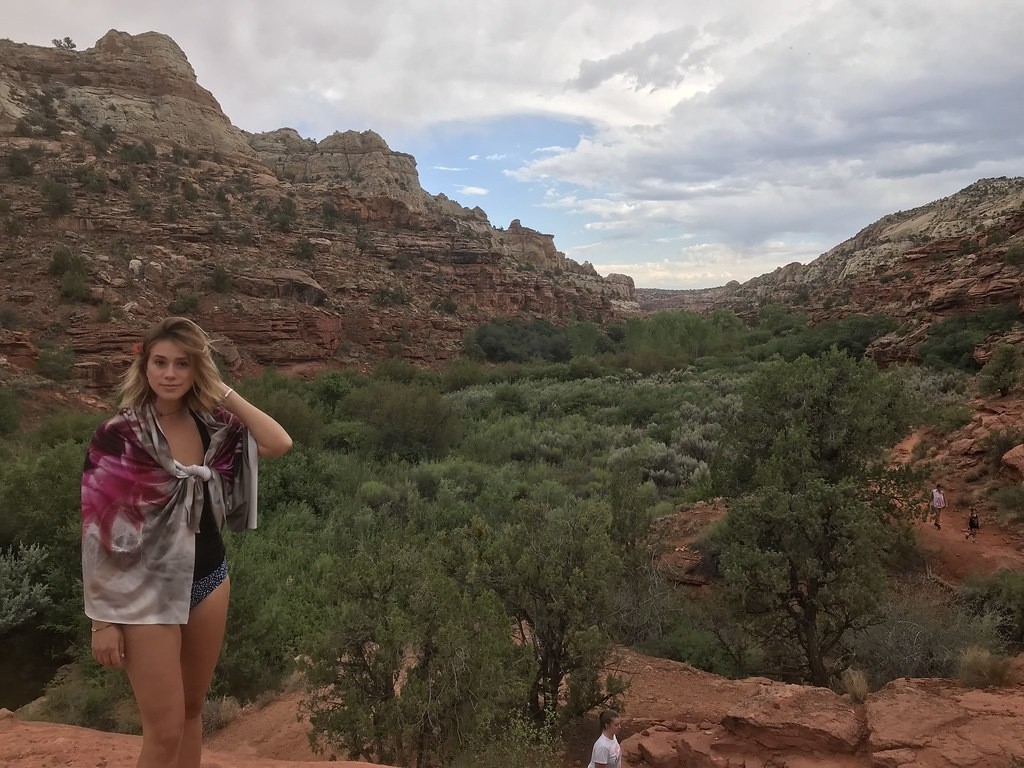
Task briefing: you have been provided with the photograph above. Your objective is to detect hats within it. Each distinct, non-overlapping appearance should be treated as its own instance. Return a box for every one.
[969,506,976,512]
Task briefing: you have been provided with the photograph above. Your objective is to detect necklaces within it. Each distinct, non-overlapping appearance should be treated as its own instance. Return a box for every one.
[155,407,184,416]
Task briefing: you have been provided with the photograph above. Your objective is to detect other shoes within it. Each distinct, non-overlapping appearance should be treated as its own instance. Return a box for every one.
[934,523,941,529]
[966,533,969,539]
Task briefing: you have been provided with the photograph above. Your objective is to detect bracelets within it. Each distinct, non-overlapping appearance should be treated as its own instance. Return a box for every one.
[91,624,112,632]
[225,388,233,397]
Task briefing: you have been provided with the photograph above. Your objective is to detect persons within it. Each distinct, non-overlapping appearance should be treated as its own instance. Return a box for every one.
[591,710,622,768]
[927,485,946,529]
[83,317,292,768]
[966,507,979,542]
